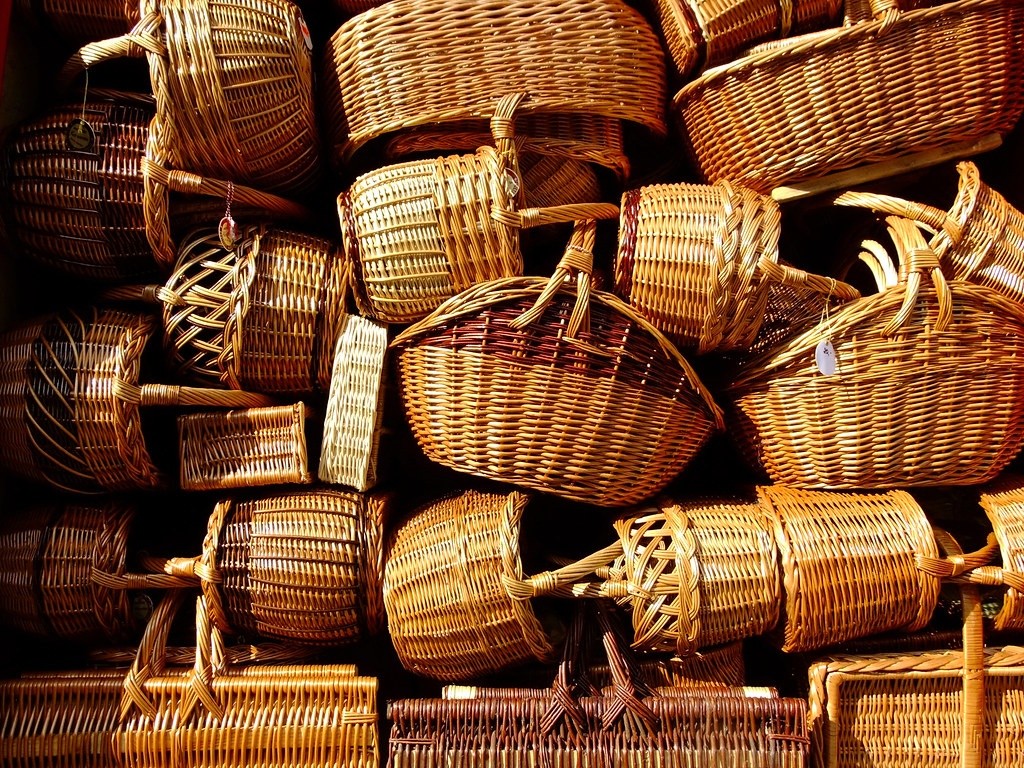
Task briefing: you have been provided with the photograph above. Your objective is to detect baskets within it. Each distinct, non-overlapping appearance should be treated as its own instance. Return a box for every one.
[0,1,1024,768]
